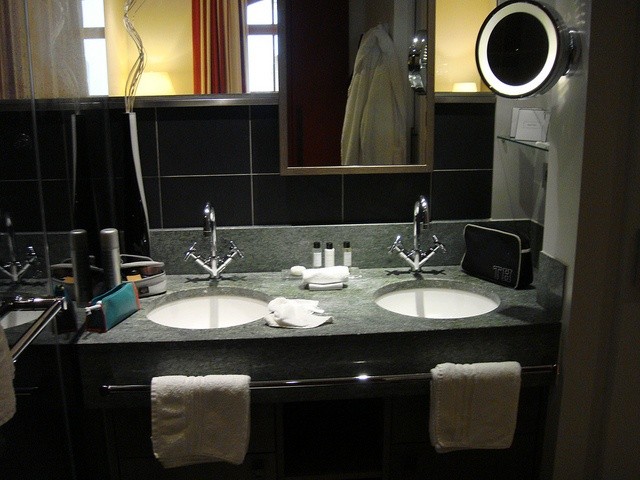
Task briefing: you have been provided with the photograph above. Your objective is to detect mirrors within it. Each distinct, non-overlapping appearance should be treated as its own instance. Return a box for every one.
[276,0,436,175]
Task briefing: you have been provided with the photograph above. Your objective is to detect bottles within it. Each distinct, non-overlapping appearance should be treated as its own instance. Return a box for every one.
[311,241,324,269]
[323,242,336,268]
[341,240,353,268]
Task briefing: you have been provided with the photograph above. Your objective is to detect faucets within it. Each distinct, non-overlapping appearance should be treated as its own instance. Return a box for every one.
[412,193,430,275]
[0,215,38,286]
[202,198,216,283]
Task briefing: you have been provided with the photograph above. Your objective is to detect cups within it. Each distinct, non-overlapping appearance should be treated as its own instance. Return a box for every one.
[98,228,122,292]
[69,229,94,308]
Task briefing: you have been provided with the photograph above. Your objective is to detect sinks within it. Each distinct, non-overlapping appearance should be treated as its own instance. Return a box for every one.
[370,279,501,320]
[145,286,272,328]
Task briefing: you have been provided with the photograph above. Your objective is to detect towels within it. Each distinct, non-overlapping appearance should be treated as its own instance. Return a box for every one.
[428,361,521,452]
[1,326,18,426]
[149,374,251,467]
[265,296,334,329]
[340,22,417,167]
